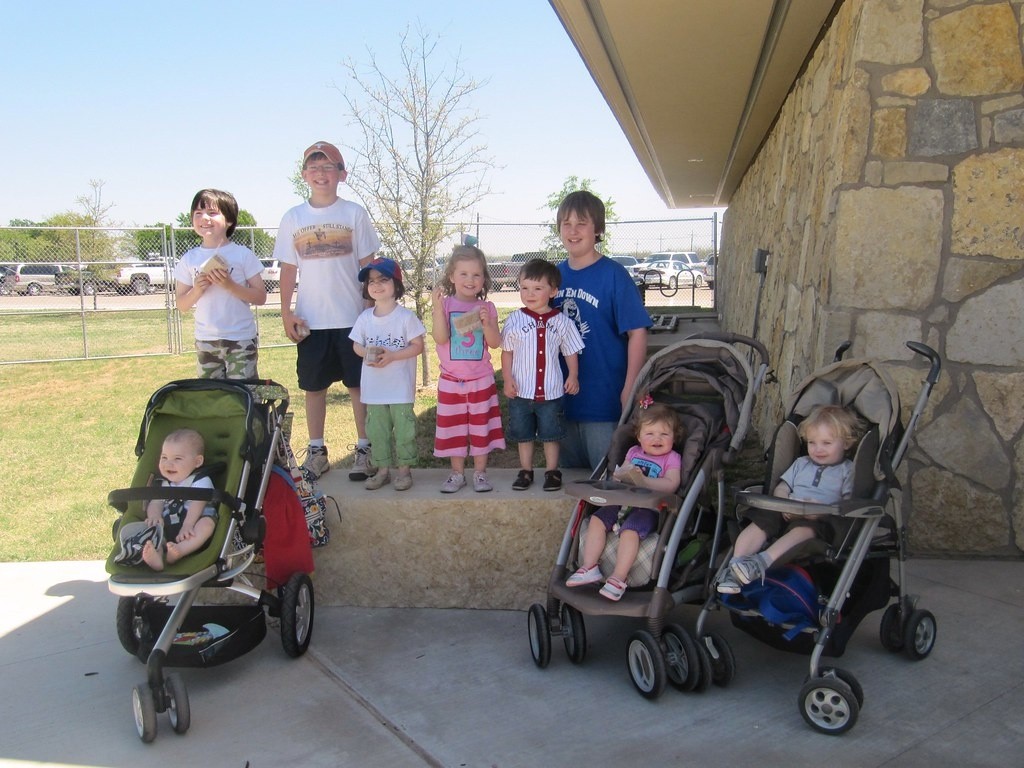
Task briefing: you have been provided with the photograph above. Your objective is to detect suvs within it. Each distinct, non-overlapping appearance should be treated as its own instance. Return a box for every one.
[632,251,703,276]
[258,257,299,293]
[704,253,719,290]
[610,255,640,279]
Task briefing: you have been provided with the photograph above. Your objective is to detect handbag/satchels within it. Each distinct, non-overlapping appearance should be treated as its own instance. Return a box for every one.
[274,429,333,546]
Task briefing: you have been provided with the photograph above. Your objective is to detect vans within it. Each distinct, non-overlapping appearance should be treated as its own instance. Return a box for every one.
[7,264,79,295]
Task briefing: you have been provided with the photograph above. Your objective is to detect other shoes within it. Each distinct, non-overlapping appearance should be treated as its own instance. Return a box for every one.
[599,575,626,602]
[565,566,603,587]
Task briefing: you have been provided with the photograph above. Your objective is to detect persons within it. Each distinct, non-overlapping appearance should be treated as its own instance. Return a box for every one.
[348,258,426,491]
[716,405,867,594]
[432,234,506,493]
[548,191,655,472]
[142,428,218,570]
[497,259,584,491]
[172,188,267,381]
[565,391,684,601]
[272,141,383,482]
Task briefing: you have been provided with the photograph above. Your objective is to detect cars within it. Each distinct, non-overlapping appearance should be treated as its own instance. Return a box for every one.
[0,266,17,295]
[399,258,443,291]
[638,261,704,290]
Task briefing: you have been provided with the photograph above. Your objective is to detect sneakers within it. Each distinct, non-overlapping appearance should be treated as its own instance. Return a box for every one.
[731,557,769,582]
[347,442,375,481]
[543,471,562,491]
[473,470,492,491]
[717,564,741,595]
[395,472,411,490]
[441,475,464,494]
[296,446,329,480]
[513,469,533,489]
[365,474,390,490]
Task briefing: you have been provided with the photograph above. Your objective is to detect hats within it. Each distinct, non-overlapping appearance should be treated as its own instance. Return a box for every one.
[358,256,402,282]
[302,141,345,170]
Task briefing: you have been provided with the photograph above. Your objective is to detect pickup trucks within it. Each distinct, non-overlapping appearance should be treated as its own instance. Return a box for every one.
[53,263,136,296]
[102,255,178,295]
[485,251,568,292]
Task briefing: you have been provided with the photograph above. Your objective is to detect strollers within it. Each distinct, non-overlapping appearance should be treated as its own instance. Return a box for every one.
[105,375,315,744]
[528,329,773,703]
[694,342,942,734]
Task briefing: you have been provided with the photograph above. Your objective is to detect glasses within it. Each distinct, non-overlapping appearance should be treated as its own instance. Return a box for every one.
[305,164,338,174]
